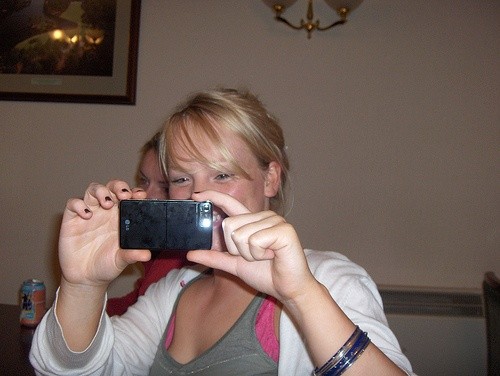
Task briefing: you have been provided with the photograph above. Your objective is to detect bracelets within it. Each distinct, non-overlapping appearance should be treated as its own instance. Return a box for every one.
[313,325,371,376]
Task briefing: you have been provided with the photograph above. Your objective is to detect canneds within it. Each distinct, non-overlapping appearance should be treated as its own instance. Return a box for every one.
[19,279,46,328]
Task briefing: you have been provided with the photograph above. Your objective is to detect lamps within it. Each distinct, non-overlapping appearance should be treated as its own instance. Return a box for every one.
[261,0,363,40]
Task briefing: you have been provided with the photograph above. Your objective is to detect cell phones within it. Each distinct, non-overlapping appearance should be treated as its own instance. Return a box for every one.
[118,199,213,250]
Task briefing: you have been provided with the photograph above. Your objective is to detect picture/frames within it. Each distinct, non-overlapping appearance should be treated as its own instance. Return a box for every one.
[0,0,142,105]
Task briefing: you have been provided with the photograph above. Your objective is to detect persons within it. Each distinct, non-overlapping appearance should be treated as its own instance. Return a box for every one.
[107,129,197,317]
[29,89,418,376]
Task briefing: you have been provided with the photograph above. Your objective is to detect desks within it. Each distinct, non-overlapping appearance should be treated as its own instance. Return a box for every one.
[0,304,36,376]
